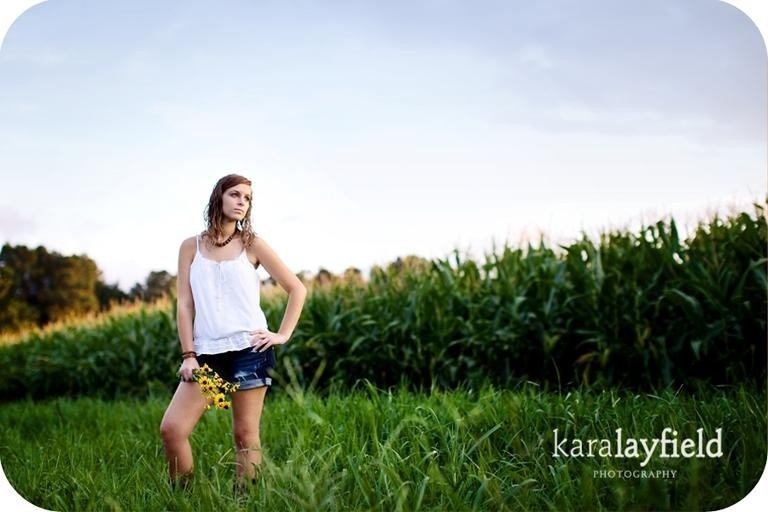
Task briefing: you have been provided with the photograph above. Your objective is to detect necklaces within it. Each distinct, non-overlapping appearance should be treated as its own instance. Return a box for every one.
[208,228,239,247]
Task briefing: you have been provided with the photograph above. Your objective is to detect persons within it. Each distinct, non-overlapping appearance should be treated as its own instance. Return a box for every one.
[160,175,307,495]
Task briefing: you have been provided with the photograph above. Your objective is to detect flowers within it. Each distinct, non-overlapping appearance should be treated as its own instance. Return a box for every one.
[177,359,241,412]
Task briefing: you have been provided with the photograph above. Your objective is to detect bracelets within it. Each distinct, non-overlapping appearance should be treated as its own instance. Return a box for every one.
[182,350,199,357]
